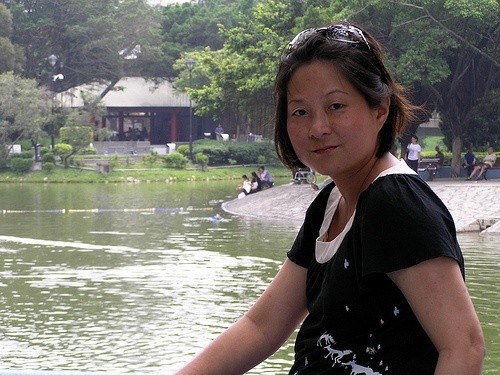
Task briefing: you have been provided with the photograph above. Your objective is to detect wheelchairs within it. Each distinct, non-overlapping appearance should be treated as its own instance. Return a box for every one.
[293,167,316,185]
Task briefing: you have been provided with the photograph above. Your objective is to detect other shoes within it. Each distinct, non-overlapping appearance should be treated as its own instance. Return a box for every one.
[465,177,473,181]
[474,177,482,181]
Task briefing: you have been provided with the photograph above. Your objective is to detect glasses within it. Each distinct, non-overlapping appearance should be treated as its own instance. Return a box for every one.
[282,24,374,60]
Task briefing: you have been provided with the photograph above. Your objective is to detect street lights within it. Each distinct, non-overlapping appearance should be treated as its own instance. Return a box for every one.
[47,52,59,151]
[184,59,196,160]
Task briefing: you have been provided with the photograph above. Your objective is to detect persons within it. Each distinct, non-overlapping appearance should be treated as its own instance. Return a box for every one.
[293,167,311,186]
[236,165,273,196]
[173,25,487,375]
[123,126,147,141]
[406,135,421,173]
[425,145,445,181]
[463,145,476,180]
[465,144,497,180]
[215,122,224,141]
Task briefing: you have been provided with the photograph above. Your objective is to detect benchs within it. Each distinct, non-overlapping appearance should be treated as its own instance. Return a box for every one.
[471,157,500,180]
[417,168,437,182]
